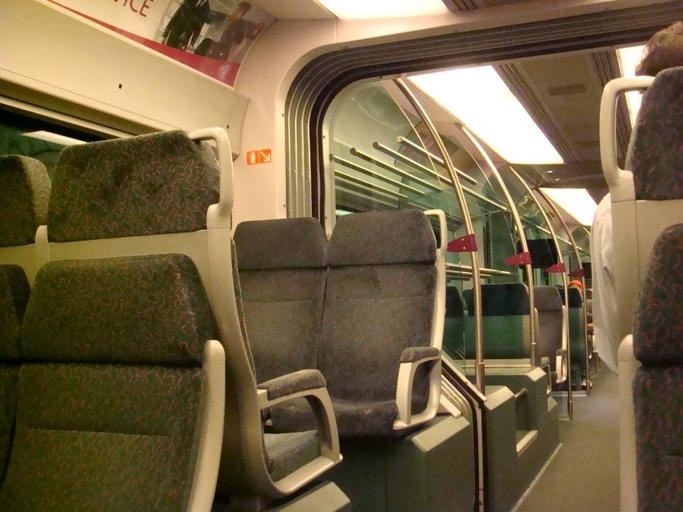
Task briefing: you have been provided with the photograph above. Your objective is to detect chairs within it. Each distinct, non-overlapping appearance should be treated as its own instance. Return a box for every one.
[2,126,448,512]
[448,283,599,512]
[600,74,683,510]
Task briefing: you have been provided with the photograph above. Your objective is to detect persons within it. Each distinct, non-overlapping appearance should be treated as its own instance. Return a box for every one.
[193,1,253,62]
[567,278,594,390]
[159,0,213,54]
[584,19,683,376]
